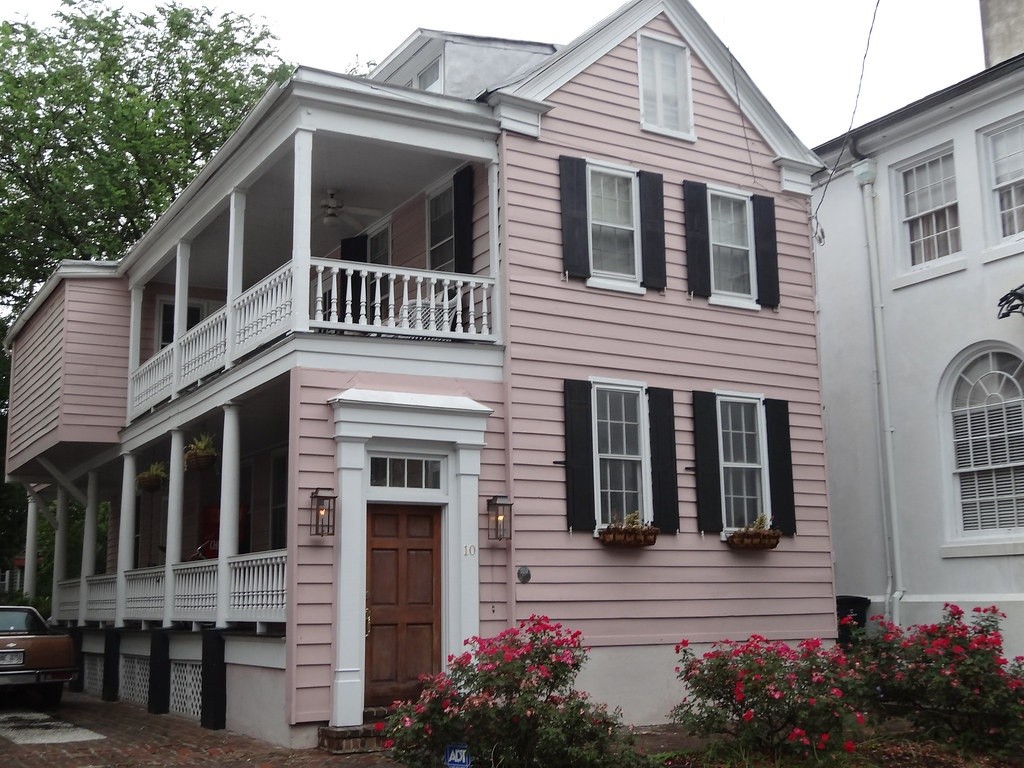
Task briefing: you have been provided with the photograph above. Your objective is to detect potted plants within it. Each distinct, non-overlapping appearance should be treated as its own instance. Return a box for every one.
[183,432,218,472]
[135,461,170,493]
[603,513,778,543]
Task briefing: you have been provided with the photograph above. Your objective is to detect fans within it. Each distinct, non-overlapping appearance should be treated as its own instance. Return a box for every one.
[283,189,383,232]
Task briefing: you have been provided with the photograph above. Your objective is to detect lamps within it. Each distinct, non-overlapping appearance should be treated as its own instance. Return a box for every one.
[487,496,514,540]
[309,488,338,537]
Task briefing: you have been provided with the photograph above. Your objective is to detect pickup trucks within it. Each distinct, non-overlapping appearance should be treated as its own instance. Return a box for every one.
[0,603,76,709]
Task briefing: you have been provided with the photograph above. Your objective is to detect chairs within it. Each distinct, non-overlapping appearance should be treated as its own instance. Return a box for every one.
[396,289,464,342]
